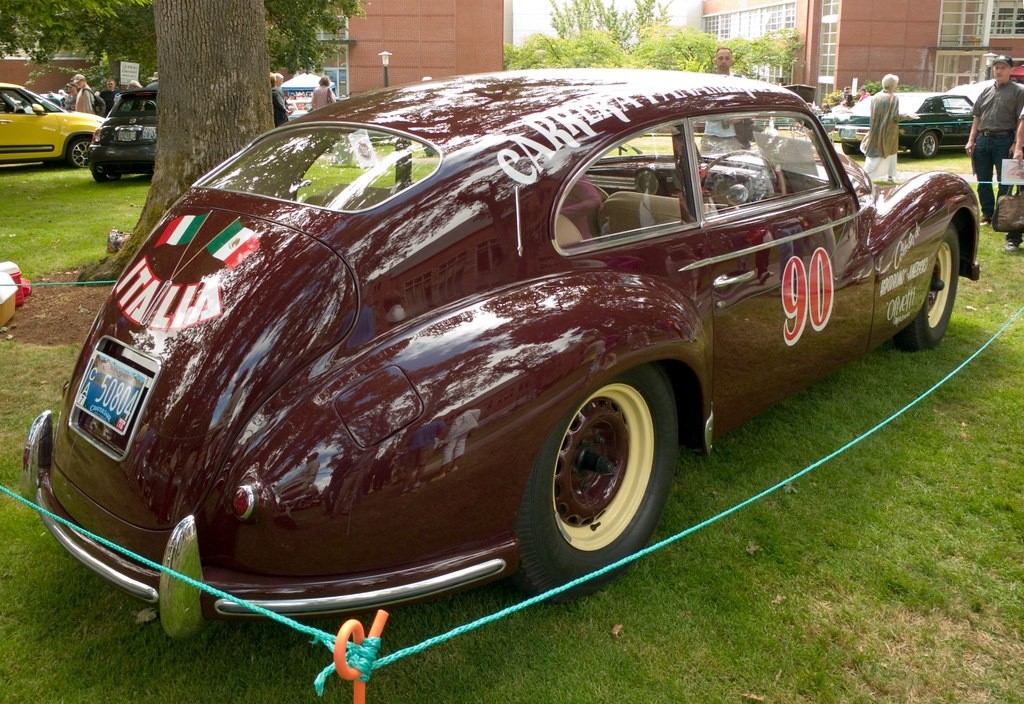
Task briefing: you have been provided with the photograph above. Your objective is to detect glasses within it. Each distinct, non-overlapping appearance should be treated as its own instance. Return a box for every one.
[66,86,71,88]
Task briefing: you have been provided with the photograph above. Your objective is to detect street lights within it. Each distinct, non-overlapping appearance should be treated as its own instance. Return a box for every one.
[378,51,393,88]
[983,51,998,80]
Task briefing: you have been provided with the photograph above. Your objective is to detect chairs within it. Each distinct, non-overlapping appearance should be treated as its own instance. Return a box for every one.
[317,181,389,212]
[554,215,583,248]
[598,190,714,230]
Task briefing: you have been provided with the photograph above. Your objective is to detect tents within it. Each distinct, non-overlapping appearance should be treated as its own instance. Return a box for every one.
[282,73,336,95]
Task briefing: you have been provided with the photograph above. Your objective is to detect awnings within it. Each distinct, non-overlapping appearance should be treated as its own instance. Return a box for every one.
[1009,67,1024,77]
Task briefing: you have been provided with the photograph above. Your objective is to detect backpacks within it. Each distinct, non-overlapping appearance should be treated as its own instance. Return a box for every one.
[82,88,106,116]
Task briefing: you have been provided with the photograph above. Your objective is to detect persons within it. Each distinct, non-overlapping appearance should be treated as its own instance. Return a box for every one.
[64,82,79,110]
[1002,108,1024,250]
[700,48,748,152]
[859,89,868,102]
[270,72,295,126]
[840,87,856,104]
[48,91,61,106]
[312,76,336,110]
[965,55,1024,226]
[58,89,67,106]
[100,80,120,116]
[73,74,95,114]
[864,74,921,180]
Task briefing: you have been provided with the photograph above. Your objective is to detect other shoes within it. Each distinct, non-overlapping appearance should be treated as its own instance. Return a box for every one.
[1004,236,1019,251]
[980,214,990,226]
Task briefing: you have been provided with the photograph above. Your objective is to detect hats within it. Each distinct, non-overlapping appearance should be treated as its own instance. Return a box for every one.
[152,72,158,80]
[992,54,1013,67]
[73,74,85,82]
[66,81,76,87]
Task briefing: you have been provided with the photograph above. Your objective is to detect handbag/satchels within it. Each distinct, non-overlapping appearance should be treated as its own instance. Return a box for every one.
[327,88,332,104]
[992,185,1024,233]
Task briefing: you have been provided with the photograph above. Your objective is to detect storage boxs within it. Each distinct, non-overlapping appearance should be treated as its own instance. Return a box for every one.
[0,272,19,328]
[0,261,25,306]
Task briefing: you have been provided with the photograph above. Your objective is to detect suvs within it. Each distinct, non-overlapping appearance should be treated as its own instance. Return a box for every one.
[88,87,158,183]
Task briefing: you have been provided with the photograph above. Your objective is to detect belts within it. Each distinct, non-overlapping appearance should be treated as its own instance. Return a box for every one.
[978,130,1011,137]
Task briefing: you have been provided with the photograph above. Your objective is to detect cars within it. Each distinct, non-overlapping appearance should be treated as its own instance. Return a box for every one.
[818,92,974,161]
[0,83,107,168]
[20,66,981,646]
